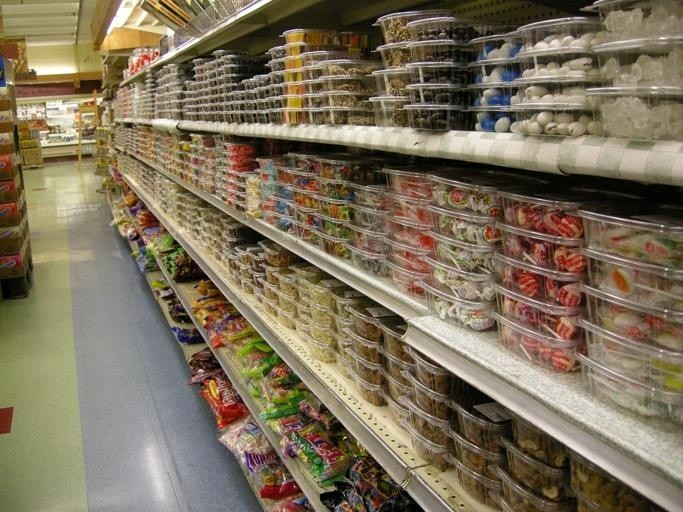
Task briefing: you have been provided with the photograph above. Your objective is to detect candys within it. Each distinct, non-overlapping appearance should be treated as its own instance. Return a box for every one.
[390,0,683,422]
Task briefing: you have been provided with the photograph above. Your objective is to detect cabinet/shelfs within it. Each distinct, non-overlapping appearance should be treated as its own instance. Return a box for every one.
[101,0,683,512]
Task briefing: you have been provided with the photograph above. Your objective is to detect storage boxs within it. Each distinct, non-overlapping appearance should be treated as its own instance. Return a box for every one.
[0,56,44,280]
